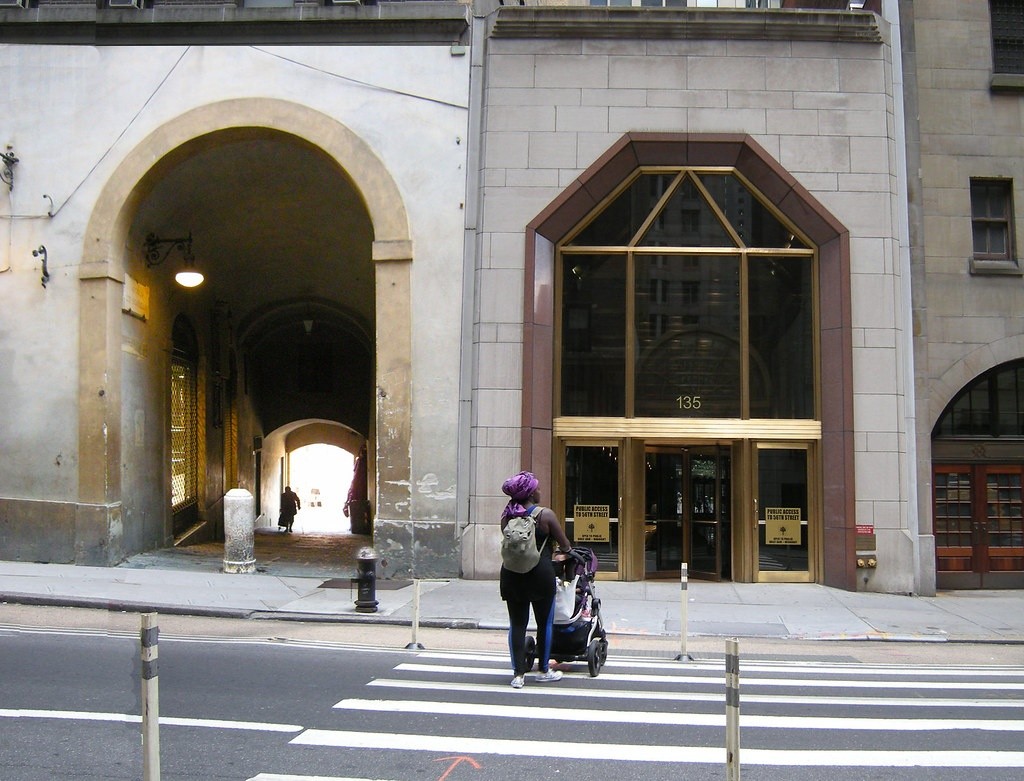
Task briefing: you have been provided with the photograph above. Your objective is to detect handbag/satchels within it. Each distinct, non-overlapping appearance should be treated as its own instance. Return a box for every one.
[554,574,579,622]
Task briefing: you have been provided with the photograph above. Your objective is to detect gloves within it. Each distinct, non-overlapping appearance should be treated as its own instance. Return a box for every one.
[564,547,586,567]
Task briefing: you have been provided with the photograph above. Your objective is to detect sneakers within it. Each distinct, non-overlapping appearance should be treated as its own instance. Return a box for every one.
[535,668,563,682]
[511,676,525,688]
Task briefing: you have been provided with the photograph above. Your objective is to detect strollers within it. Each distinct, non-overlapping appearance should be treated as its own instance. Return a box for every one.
[522,546,608,677]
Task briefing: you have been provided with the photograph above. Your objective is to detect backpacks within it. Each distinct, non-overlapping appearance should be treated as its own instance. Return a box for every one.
[501,507,549,573]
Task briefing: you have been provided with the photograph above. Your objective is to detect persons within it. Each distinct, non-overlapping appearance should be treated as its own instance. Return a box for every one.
[499,471,587,688]
[554,553,584,605]
[280,486,301,533]
